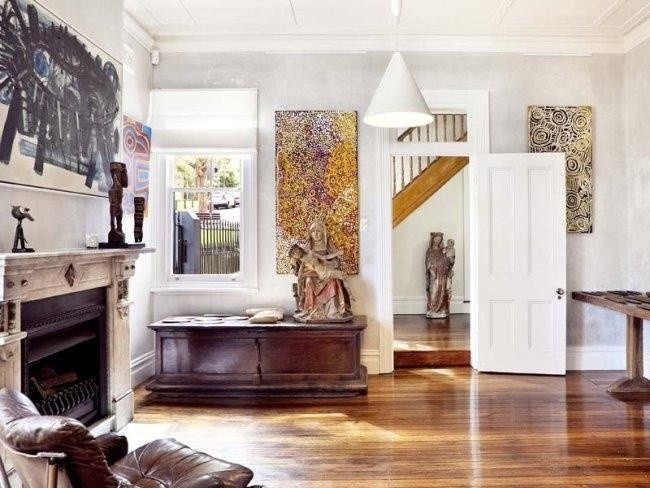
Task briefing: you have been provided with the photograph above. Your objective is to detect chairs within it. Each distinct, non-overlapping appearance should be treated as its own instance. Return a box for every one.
[0,387,262,488]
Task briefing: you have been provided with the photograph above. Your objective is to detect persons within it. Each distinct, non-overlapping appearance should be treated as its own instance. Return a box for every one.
[293,221,350,322]
[289,244,343,281]
[424,232,449,313]
[443,239,456,266]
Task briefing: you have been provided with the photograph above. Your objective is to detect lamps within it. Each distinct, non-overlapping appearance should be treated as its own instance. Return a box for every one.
[362,0,434,129]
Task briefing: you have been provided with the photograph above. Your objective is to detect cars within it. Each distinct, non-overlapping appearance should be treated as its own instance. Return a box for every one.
[208,186,241,208]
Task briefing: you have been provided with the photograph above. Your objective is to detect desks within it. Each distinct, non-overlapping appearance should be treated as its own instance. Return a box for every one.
[139,314,369,407]
[571,290,650,402]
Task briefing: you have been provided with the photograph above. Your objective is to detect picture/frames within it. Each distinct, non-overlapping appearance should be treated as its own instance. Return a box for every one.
[0,0,123,199]
